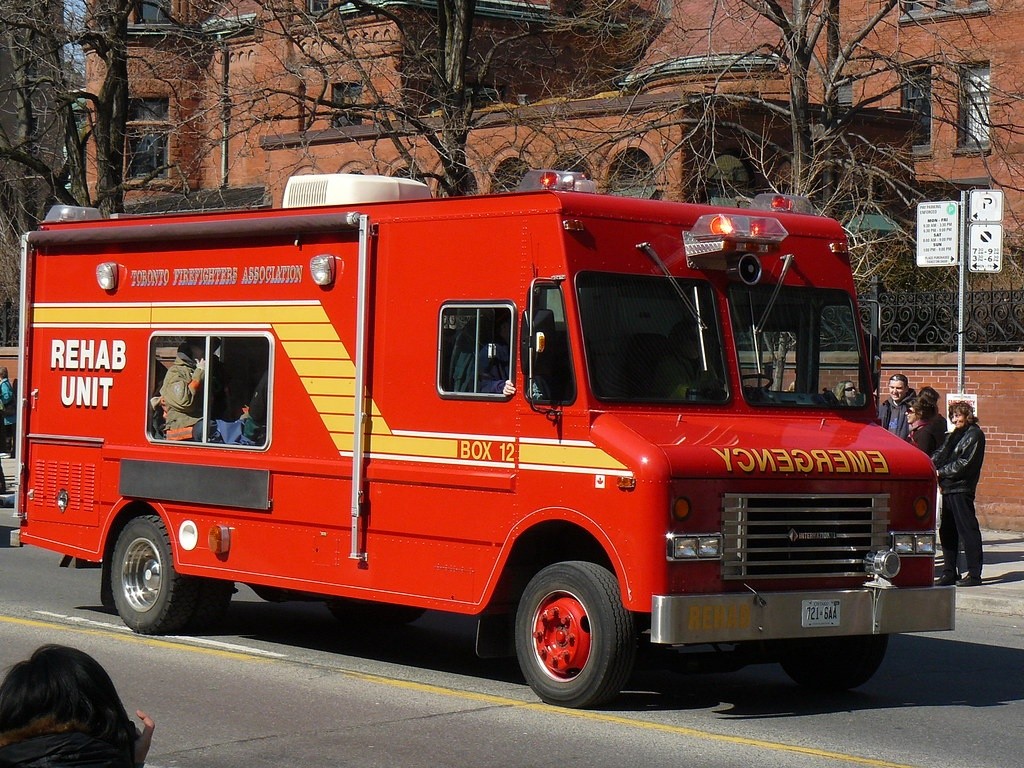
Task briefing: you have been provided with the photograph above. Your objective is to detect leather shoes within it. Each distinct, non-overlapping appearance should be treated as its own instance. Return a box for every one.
[934,575,961,586]
[956,575,982,587]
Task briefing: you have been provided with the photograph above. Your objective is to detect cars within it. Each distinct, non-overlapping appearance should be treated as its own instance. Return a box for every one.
[0,490,15,507]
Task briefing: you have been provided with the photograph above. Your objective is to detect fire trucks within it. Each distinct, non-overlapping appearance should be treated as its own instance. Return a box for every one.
[10,167,953,707]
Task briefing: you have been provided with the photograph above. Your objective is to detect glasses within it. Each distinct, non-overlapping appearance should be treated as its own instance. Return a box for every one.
[907,409,915,413]
[845,386,855,392]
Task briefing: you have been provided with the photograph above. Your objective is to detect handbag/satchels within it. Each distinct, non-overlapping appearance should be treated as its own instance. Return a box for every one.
[0,383,16,417]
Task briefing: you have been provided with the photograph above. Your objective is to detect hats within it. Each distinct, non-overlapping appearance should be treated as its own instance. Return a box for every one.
[177,336,221,353]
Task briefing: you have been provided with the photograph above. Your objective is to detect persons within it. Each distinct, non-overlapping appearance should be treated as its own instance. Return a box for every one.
[932,401,986,587]
[159,338,205,442]
[634,312,727,402]
[0,367,17,459]
[472,317,516,396]
[784,379,796,393]
[0,643,155,768]
[878,374,916,444]
[832,380,866,407]
[905,386,948,460]
[210,348,268,445]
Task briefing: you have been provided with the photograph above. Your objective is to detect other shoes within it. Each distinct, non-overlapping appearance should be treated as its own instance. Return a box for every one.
[0,452,11,459]
